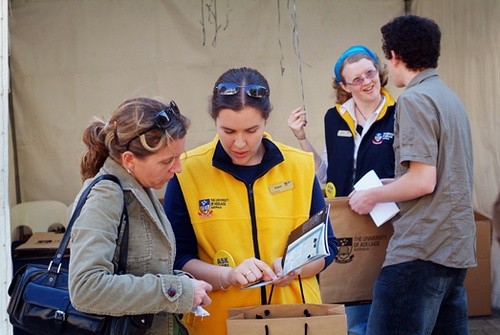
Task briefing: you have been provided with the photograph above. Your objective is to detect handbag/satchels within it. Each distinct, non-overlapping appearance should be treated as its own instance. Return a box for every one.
[7,263,153,335]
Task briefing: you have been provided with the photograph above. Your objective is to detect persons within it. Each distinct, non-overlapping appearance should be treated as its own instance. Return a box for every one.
[163,69,338,335]
[69,98,212,335]
[285,44,397,198]
[348,14,478,335]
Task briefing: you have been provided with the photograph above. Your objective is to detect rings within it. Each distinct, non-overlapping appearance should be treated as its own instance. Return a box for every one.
[244,271,252,277]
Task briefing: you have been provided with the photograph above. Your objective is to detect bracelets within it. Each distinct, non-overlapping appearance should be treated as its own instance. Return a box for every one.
[296,136,306,140]
[217,267,232,291]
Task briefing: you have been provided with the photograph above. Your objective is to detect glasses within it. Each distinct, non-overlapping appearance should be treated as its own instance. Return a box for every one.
[214,82,268,99]
[344,64,378,87]
[126,100,180,152]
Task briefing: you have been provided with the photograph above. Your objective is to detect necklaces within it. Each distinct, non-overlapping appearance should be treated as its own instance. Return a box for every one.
[354,97,383,121]
